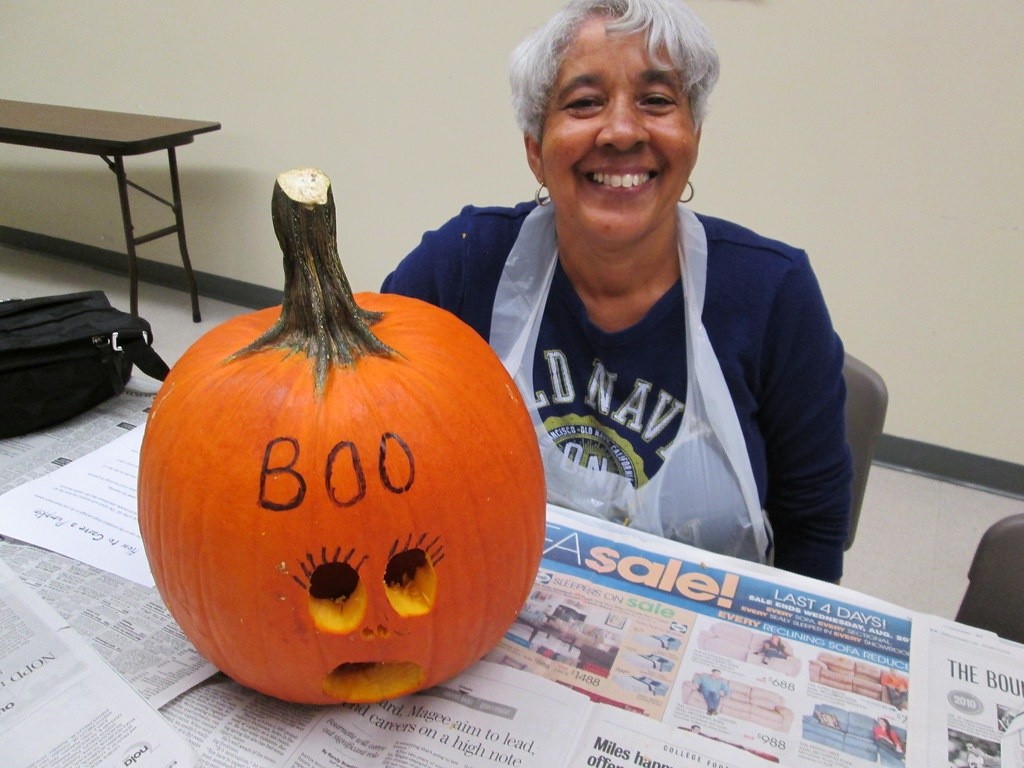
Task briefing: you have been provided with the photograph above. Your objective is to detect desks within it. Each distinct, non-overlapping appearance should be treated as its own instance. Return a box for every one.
[0,96,224,328]
[2,353,1024,767]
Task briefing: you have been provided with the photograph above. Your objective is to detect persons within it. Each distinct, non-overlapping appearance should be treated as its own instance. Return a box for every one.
[881,666,910,709]
[695,666,730,716]
[959,742,994,768]
[874,718,905,767]
[754,634,791,667]
[691,725,701,734]
[375,0,856,589]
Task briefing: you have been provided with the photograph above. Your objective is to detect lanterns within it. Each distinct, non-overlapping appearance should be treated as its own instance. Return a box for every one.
[136,166,550,708]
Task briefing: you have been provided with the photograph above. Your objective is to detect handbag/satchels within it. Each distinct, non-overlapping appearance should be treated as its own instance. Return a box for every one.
[0,289,173,439]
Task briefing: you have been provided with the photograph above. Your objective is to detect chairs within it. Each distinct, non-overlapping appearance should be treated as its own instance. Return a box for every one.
[843,354,888,603]
[979,615,985,618]
[953,512,1024,647]
